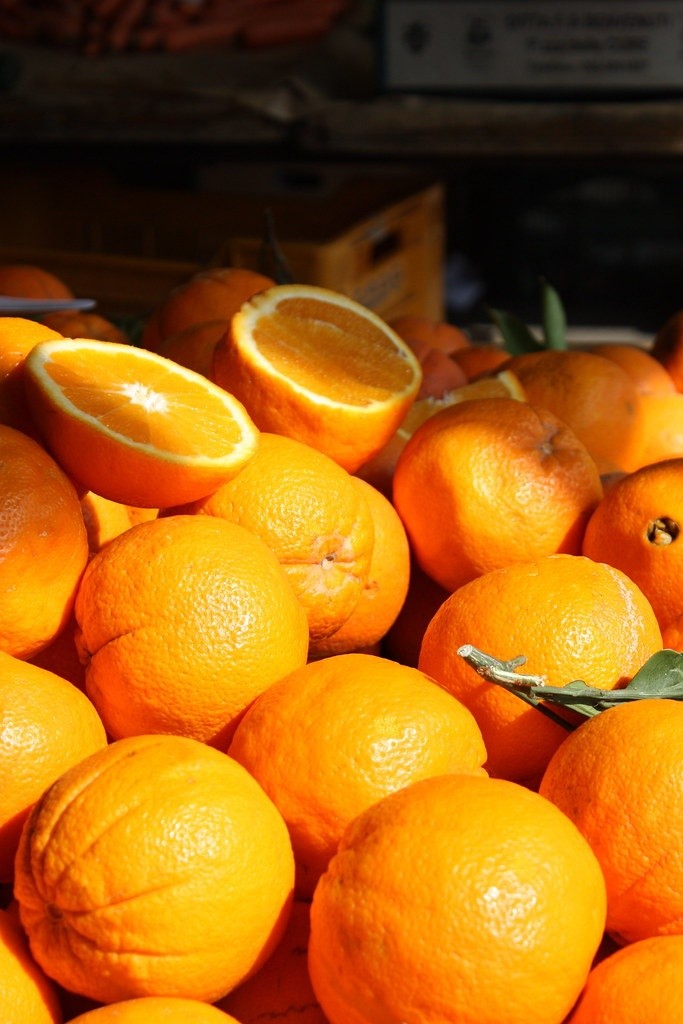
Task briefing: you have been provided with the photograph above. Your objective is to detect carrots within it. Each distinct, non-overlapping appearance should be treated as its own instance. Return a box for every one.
[0,0,351,51]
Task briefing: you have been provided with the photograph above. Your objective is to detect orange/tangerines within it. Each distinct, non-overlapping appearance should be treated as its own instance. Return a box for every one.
[0,267,683,1024]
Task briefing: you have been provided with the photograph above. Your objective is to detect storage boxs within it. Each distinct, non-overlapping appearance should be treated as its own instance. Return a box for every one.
[0,161,444,328]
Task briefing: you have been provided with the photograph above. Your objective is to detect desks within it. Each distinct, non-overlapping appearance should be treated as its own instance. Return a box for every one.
[0,91,683,329]
